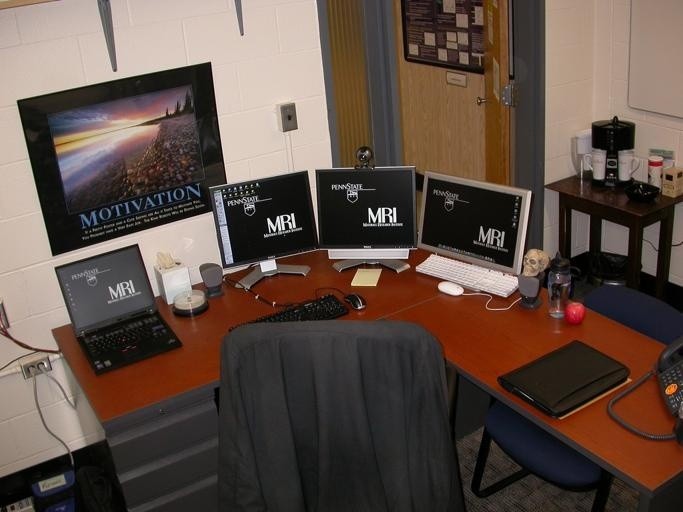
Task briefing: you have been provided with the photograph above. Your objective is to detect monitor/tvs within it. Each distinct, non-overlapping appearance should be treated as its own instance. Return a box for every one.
[315,166,417,274]
[417,171,533,275]
[209,170,319,289]
[17,62,227,257]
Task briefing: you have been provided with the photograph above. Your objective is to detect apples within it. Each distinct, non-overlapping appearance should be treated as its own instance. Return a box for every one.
[565,302,586,325]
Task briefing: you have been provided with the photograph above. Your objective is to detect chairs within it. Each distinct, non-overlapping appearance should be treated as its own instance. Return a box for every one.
[468,281,683,510]
[216,316,454,512]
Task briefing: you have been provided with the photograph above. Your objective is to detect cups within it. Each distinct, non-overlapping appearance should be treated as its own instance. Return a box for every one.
[619,150,640,182]
[584,150,606,180]
[647,156,663,187]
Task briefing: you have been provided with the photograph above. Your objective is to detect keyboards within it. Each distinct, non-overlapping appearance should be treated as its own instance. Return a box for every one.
[415,254,519,298]
[229,293,349,333]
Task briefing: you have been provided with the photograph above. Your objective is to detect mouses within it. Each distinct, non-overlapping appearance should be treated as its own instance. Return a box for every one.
[345,295,366,310]
[438,281,464,296]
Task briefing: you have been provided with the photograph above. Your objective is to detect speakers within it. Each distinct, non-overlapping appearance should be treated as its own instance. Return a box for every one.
[199,263,223,297]
[519,276,543,308]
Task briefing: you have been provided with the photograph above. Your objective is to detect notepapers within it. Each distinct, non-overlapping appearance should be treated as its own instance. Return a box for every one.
[350,268,384,287]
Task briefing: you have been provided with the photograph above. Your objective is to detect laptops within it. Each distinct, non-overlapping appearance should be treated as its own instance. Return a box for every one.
[54,244,182,375]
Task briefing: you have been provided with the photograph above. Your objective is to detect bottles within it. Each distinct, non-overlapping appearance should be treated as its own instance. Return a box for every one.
[547,252,582,318]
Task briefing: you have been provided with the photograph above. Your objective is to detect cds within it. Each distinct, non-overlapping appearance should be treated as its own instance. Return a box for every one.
[172,290,208,317]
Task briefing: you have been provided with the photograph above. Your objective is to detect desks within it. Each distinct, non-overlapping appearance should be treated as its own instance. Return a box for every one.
[48,246,683,511]
[544,170,682,301]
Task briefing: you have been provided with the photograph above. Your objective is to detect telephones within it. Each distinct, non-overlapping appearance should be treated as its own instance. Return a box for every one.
[656,336,683,416]
